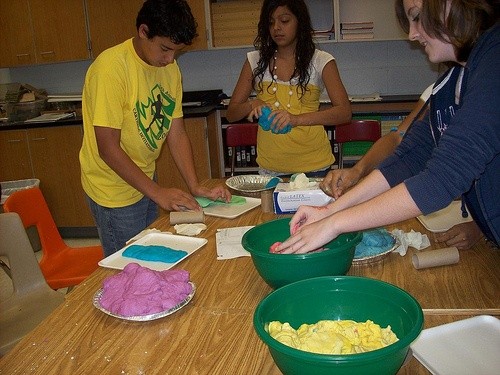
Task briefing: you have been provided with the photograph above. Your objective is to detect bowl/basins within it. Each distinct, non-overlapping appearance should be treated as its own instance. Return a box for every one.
[241,217,363,289]
[252,276,425,375]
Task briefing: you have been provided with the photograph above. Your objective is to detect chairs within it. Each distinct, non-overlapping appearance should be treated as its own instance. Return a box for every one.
[3,187,104,290]
[226,123,258,177]
[335,120,381,169]
[0,212,64,358]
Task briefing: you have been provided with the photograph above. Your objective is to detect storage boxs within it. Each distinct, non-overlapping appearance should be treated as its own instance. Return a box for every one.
[0,97,49,122]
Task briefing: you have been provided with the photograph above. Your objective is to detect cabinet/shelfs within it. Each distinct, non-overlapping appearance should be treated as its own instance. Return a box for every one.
[0,0,90,68]
[0,107,99,239]
[156,105,220,217]
[206,0,409,50]
[84,0,208,59]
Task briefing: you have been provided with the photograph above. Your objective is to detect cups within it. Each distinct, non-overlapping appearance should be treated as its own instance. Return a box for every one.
[261,190,273,213]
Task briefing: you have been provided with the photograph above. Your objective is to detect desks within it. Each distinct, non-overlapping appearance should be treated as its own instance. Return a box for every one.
[64,178,500,315]
[0,301,500,375]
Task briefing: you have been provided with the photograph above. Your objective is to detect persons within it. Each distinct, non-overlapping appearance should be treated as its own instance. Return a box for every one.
[318,82,483,250]
[275,0,500,254]
[79,0,233,258]
[227,0,351,178]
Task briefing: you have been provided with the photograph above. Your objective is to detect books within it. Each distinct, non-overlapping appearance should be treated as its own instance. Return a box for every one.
[340,21,374,39]
[311,30,331,40]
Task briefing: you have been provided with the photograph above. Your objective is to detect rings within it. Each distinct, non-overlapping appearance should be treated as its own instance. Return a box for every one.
[325,187,329,191]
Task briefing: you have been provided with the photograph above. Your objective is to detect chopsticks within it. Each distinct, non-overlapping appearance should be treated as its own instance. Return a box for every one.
[421,308,500,315]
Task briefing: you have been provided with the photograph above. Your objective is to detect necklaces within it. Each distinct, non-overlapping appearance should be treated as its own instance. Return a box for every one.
[273,49,293,110]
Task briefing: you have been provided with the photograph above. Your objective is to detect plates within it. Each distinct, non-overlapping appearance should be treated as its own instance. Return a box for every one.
[97,233,208,272]
[187,197,261,218]
[352,231,402,264]
[409,315,500,375]
[93,281,197,321]
[416,199,474,232]
[226,175,284,196]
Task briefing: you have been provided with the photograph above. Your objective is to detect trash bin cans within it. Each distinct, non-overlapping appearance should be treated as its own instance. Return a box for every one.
[0,179,42,252]
